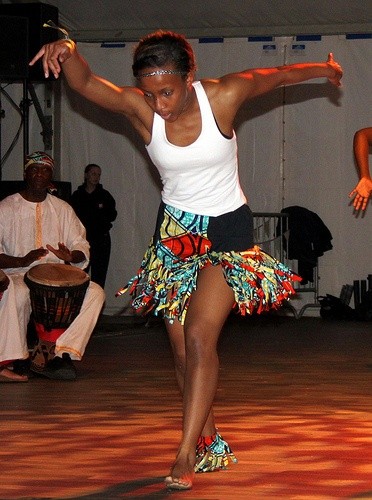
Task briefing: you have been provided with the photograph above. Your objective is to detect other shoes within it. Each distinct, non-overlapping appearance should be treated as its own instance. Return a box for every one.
[0,369,28,382]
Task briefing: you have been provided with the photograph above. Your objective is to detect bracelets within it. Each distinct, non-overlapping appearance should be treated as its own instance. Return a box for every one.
[43,20,75,52]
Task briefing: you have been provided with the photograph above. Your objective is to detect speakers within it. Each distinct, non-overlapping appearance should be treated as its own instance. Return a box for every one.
[0,1,59,84]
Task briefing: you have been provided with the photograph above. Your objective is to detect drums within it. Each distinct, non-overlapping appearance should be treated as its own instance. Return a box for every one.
[22,261,90,377]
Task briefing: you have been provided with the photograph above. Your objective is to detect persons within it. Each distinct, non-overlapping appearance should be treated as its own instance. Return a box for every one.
[348,127,372,211]
[0,150,108,382]
[29,31,346,491]
[68,163,118,337]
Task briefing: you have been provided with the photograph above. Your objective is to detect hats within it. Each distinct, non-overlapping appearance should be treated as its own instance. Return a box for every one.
[24,151,56,173]
